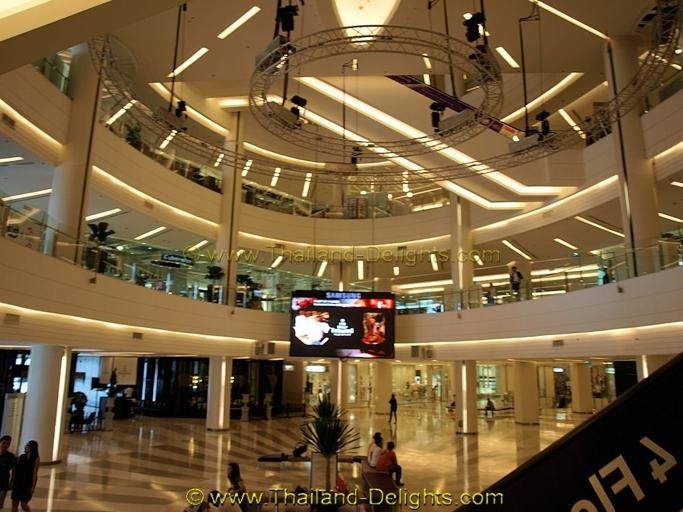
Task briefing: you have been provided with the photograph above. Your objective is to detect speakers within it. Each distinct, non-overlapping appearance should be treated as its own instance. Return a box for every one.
[409,346,423,359]
[265,343,275,354]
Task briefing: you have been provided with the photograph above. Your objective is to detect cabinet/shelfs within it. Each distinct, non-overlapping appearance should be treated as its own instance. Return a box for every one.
[98,397,136,420]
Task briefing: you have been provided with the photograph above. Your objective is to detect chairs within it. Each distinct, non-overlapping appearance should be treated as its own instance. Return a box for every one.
[84,411,96,430]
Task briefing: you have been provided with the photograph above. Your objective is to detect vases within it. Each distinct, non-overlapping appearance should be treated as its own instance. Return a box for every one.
[64,398,74,434]
[242,394,250,421]
[264,393,273,420]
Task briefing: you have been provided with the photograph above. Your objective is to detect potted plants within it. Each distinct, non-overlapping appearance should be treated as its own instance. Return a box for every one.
[87,221,116,274]
[306,388,361,508]
[204,265,253,308]
[104,368,116,431]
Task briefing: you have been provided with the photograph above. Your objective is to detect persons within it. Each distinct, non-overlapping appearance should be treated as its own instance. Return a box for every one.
[108,367,118,387]
[0,435,16,510]
[601,267,610,284]
[366,432,382,461]
[205,490,242,511]
[485,283,496,304]
[13,438,40,511]
[388,424,397,449]
[368,436,383,468]
[374,440,404,486]
[509,264,523,300]
[482,397,495,415]
[225,461,247,509]
[386,393,397,423]
[447,394,456,412]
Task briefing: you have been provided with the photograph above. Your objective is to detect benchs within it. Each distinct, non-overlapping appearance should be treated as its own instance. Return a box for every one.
[441,405,516,419]
[258,451,402,509]
